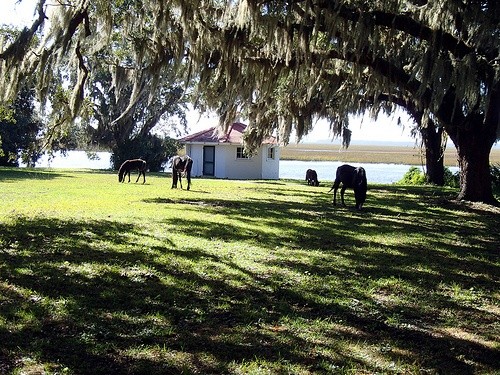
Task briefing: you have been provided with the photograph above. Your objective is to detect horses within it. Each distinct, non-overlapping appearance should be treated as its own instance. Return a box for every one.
[117,158,147,185]
[170,155,193,191]
[305,169,318,187]
[328,164,368,211]
